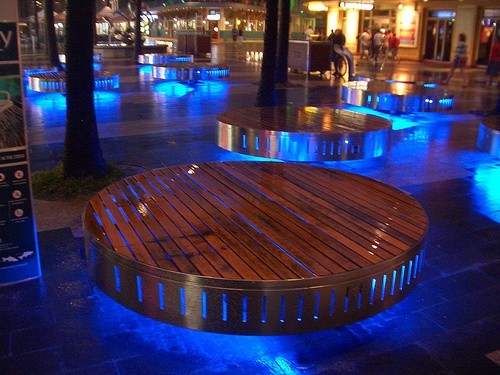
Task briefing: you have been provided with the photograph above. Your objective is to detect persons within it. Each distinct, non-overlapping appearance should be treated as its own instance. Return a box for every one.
[359,27,401,62]
[328,29,345,77]
[232,27,237,48]
[315,29,323,41]
[486,37,500,86]
[239,28,243,49]
[443,33,469,87]
[305,26,314,41]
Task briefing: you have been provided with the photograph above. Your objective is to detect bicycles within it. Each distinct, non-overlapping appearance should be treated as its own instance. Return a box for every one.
[331,49,347,76]
